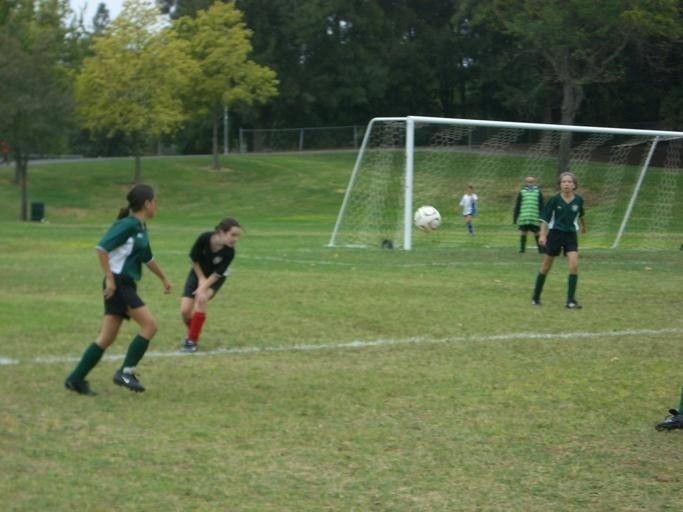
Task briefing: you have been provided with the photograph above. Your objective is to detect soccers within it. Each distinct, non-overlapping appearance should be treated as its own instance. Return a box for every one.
[413,206,442,232]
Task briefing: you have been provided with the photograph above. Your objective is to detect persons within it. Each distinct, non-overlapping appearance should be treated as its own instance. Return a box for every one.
[653,378,683,433]
[511,177,547,257]
[176,217,245,352]
[529,172,586,310]
[453,185,477,238]
[61,184,176,398]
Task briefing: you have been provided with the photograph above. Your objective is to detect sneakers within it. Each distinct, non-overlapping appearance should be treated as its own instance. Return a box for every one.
[565,300,583,309]
[654,408,683,432]
[181,338,199,352]
[113,368,148,394]
[530,298,541,305]
[64,376,99,397]
